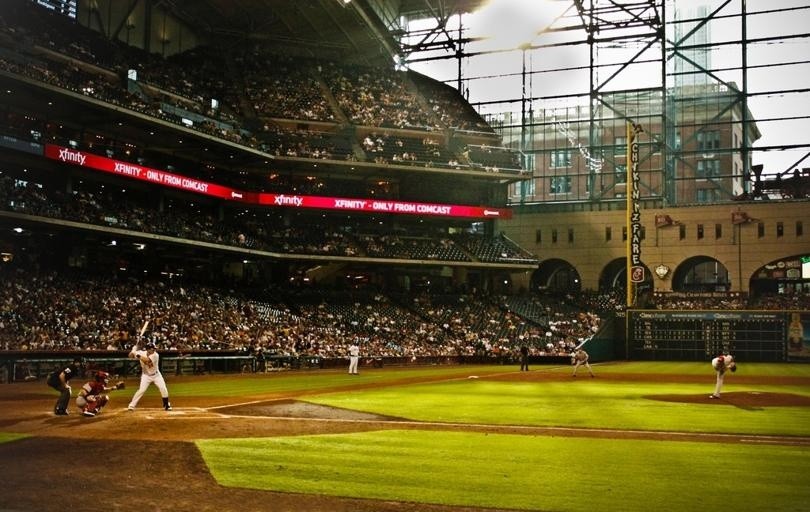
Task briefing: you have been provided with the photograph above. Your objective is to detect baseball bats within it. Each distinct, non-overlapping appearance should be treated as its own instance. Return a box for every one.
[134,321,150,345]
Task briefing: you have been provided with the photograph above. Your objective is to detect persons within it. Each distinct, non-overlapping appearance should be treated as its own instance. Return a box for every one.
[707,353,736,399]
[122,341,172,410]
[45,356,90,416]
[75,370,125,417]
[1,1,608,384]
[609,281,810,318]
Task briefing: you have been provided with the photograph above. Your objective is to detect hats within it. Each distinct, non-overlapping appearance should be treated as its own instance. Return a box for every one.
[144,341,159,350]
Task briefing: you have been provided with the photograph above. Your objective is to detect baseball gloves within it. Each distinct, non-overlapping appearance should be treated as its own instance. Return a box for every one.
[117,382,125,389]
[730,363,736,372]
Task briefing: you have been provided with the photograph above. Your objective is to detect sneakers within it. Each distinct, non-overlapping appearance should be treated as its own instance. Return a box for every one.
[53,406,100,417]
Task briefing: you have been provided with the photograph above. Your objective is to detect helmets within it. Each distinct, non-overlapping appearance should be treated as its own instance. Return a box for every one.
[94,371,110,383]
